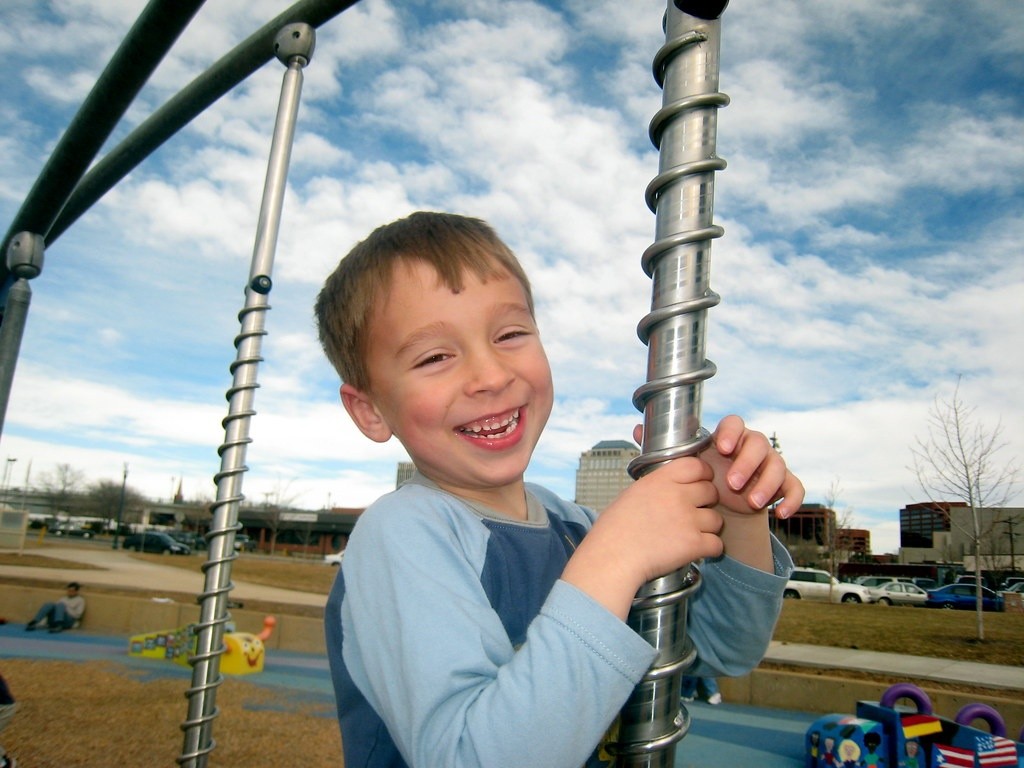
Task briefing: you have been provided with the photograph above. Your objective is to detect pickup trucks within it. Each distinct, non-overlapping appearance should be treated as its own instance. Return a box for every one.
[853,574,935,589]
[233,535,258,553]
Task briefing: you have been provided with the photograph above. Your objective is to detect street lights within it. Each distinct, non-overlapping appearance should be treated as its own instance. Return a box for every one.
[1,458,17,481]
[113,459,131,551]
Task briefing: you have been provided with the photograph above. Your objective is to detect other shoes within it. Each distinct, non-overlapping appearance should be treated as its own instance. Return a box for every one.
[26,621,36,630]
[50,624,63,632]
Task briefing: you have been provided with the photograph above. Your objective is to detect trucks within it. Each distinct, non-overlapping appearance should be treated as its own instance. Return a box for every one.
[28,512,134,536]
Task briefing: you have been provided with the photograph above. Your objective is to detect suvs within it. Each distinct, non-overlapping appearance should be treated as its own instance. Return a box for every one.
[1002,577,1024,588]
[952,573,988,594]
[784,567,872,606]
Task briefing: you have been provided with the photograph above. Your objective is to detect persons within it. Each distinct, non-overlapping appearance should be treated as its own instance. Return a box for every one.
[944,567,956,584]
[0,674,18,768]
[25,582,86,632]
[314,212,804,768]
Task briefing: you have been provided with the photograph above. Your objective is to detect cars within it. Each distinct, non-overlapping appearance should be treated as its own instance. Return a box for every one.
[926,582,1005,612]
[324,550,346,567]
[123,532,191,556]
[867,580,927,607]
[168,530,207,552]
[49,521,95,540]
[1007,582,1024,592]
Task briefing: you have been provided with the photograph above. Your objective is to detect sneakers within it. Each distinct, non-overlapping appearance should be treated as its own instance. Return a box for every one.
[681,697,694,703]
[708,693,722,704]
